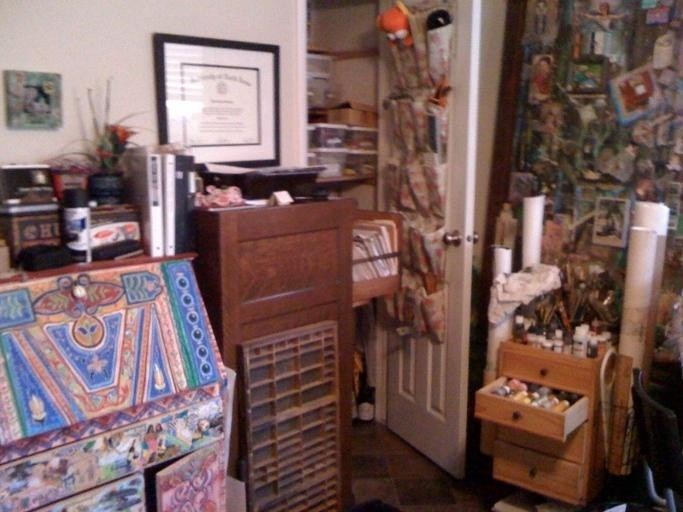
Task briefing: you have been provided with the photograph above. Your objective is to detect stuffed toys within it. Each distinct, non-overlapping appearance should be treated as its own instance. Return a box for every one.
[374,4,414,48]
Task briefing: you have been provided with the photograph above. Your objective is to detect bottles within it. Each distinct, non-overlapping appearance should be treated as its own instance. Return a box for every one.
[61,187,91,265]
[513,312,612,362]
[492,377,578,413]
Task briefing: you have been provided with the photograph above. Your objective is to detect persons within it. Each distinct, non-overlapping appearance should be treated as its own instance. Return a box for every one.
[580,3,628,30]
[23,81,52,117]
[531,55,550,96]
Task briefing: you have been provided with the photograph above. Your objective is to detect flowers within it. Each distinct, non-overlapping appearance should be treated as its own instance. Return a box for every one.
[46,76,146,173]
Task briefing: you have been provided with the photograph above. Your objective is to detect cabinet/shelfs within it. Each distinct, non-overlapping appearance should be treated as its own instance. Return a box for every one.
[186,189,357,512]
[475,333,620,512]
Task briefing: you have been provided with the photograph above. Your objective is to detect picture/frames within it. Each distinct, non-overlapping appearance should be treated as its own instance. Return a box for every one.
[4,71,60,128]
[153,32,281,168]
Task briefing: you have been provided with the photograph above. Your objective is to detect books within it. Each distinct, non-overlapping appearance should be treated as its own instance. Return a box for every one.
[353,215,401,283]
[129,152,164,263]
[173,153,189,255]
[144,152,180,258]
[188,155,196,253]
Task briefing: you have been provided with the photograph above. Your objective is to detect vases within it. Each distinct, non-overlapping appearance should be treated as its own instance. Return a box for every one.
[88,171,125,206]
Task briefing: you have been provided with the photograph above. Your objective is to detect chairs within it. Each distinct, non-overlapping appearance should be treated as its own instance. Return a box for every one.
[631,367,683,512]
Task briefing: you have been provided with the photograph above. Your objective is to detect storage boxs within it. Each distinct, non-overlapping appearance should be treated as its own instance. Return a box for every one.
[306,99,380,176]
